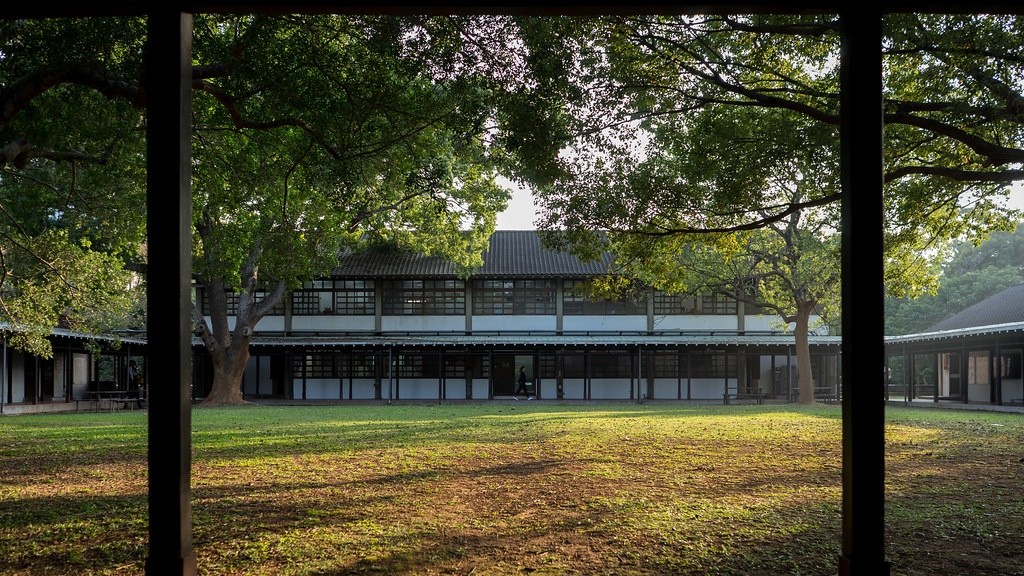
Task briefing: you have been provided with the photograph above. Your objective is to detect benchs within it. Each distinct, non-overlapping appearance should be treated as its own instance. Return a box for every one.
[73,399,137,412]
[723,394,836,405]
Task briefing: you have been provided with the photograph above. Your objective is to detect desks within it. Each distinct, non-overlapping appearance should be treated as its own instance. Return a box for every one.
[724,387,763,405]
[793,387,833,405]
[83,390,127,413]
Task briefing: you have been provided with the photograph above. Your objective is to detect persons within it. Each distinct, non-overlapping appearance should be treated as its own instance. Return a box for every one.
[124,360,144,409]
[512,366,533,401]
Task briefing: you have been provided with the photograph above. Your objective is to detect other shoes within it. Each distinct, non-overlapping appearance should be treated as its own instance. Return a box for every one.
[528,398,533,400]
[513,396,518,400]
